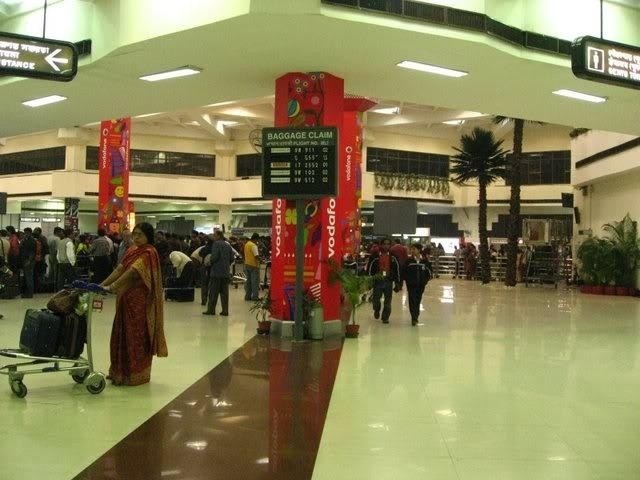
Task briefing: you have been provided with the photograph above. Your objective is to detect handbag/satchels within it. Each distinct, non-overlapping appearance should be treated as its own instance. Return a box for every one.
[46,290,78,314]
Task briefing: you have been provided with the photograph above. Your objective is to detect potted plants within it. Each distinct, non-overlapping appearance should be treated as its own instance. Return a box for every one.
[327,257,386,338]
[574,211,640,297]
[310,299,324,340]
[248,290,276,335]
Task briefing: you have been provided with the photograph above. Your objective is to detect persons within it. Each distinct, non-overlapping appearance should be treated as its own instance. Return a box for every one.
[360,238,445,328]
[95,221,168,387]
[452,242,506,280]
[1,224,272,316]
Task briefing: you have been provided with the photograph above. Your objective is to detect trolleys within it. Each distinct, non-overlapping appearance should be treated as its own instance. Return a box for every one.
[230,261,272,291]
[1,279,108,399]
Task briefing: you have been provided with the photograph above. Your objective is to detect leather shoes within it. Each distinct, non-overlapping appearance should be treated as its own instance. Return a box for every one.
[374,310,379,319]
[383,319,389,323]
[203,311,215,315]
[412,320,416,326]
[220,312,228,316]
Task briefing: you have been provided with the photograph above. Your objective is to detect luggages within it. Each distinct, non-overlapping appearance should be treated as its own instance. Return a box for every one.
[61,312,85,356]
[165,276,179,301]
[2,274,19,299]
[19,308,60,357]
